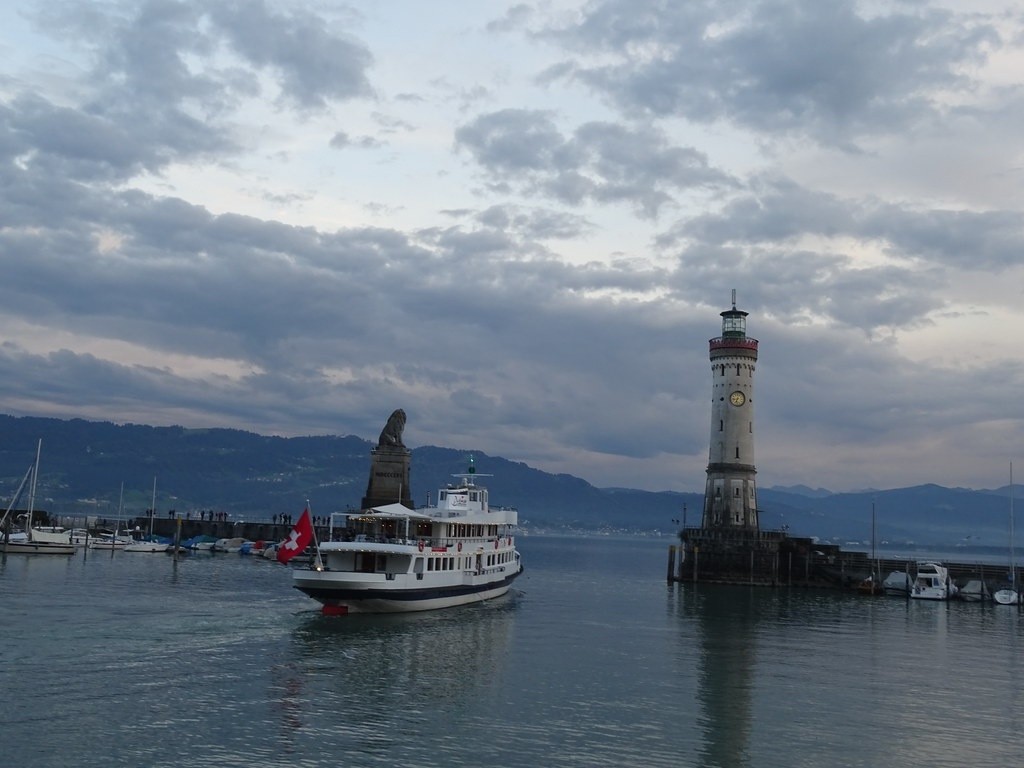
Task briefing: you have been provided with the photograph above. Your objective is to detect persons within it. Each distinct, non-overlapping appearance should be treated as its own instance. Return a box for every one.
[312,514,331,526]
[145,509,152,518]
[208,511,228,522]
[346,526,358,542]
[186,511,191,520]
[200,510,205,520]
[168,509,175,520]
[273,512,292,525]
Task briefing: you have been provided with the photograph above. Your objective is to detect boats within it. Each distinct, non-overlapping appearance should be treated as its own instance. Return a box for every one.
[210,538,327,563]
[292,447,523,616]
[910,561,959,600]
[883,571,912,597]
[191,542,214,551]
[10,526,92,543]
[961,581,990,602]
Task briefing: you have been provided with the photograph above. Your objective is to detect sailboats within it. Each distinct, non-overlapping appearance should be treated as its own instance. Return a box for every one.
[856,502,884,593]
[0,438,78,554]
[993,462,1019,605]
[123,476,169,552]
[88,480,129,549]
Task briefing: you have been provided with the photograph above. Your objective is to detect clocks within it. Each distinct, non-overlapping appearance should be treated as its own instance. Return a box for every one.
[730,391,745,407]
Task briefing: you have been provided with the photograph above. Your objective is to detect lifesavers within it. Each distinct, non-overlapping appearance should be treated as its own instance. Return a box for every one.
[495,540,498,550]
[458,542,463,553]
[418,541,424,552]
[508,538,511,546]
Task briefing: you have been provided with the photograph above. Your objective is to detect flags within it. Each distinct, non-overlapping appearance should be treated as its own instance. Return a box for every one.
[276,508,313,566]
